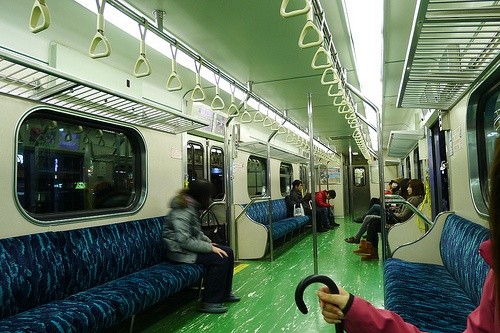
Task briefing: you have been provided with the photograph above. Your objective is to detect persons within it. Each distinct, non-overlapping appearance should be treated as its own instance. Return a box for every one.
[345,177,425,261]
[314,190,340,229]
[316,137,500,333]
[32,176,135,214]
[290,180,329,232]
[161,178,240,313]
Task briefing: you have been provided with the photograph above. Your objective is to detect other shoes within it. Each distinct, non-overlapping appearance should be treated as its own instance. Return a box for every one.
[330,222,340,226]
[318,227,328,231]
[354,215,363,223]
[324,224,335,228]
[198,302,228,312]
[224,295,242,301]
[363,234,368,238]
[345,236,360,243]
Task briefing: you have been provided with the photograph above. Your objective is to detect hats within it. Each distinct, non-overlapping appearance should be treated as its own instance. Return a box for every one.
[391,178,401,184]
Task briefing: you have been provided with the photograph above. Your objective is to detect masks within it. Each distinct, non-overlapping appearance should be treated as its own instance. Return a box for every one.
[392,182,398,188]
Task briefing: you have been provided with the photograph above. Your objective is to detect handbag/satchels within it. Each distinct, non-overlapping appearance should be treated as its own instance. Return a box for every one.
[200,209,226,245]
[293,202,305,216]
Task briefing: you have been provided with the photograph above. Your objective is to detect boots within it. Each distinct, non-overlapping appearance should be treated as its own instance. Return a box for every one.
[361,247,379,260]
[353,241,372,255]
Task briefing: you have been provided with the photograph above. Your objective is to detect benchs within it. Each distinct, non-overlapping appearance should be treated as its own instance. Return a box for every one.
[233,198,311,260]
[383,212,490,333]
[0,214,207,333]
